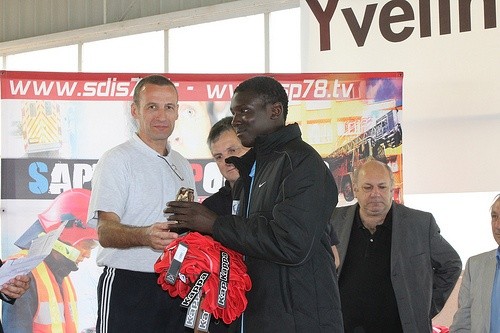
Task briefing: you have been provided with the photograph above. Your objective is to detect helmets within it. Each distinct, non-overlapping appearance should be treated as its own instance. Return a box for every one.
[37,189,99,247]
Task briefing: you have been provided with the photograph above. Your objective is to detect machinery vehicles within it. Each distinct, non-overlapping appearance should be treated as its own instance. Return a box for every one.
[320,109,403,201]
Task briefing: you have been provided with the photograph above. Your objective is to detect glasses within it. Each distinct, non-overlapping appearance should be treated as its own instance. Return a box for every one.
[82,239,97,249]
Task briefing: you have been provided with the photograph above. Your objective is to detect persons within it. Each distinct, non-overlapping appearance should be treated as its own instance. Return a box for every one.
[449,193,500,333]
[0,257,32,333]
[84,75,200,333]
[164,76,346,333]
[330,155,463,333]
[2,188,98,333]
